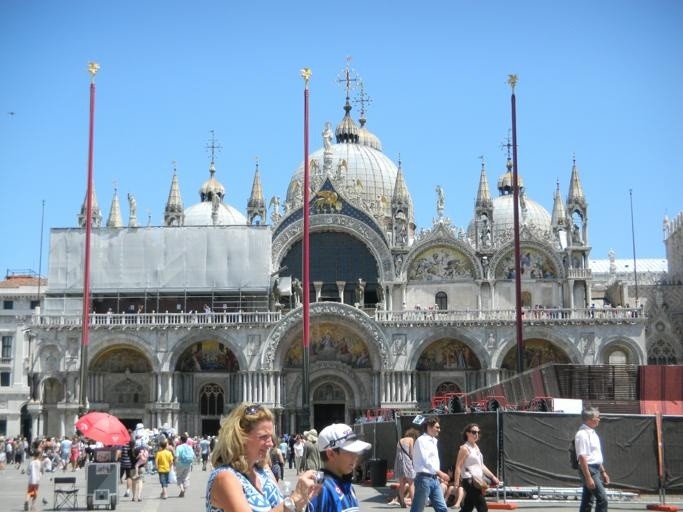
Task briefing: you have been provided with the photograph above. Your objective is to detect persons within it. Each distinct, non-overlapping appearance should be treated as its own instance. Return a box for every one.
[409,254,465,279]
[1,423,217,511]
[205,404,372,512]
[393,416,501,512]
[416,304,434,321]
[520,302,639,320]
[92,304,145,314]
[190,304,216,324]
[579,406,610,512]
[501,251,554,279]
[321,124,333,151]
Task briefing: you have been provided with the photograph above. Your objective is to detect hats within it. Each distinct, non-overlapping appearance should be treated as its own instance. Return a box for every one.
[303,429,318,442]
[136,423,144,429]
[318,424,372,453]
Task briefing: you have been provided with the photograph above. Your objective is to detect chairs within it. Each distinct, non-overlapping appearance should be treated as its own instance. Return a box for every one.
[54,476,79,511]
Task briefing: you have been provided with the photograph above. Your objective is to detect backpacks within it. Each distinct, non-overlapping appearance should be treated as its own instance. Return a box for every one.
[568,428,590,469]
[180,445,194,464]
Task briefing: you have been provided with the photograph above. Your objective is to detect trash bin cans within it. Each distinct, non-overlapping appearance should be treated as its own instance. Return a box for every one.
[368,458,388,487]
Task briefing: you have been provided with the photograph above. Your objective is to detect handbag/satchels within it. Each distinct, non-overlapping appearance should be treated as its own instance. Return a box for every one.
[473,474,492,490]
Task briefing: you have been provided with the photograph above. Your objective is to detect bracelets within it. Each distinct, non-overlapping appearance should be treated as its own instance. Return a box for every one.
[601,470,606,473]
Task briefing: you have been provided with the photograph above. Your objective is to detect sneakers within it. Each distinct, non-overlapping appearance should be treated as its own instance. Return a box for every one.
[160,493,167,499]
[124,492,142,502]
[452,506,460,509]
[132,476,139,479]
[25,502,29,510]
[180,490,185,497]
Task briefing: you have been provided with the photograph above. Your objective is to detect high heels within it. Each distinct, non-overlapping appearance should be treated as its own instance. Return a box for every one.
[400,500,406,508]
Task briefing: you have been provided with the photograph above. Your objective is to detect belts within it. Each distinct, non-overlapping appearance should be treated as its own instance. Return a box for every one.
[418,472,437,479]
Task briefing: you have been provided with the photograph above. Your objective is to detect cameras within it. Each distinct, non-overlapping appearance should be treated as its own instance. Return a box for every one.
[314,471,325,485]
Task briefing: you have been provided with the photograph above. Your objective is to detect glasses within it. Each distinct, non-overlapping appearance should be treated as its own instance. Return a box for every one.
[244,404,264,417]
[467,429,480,434]
